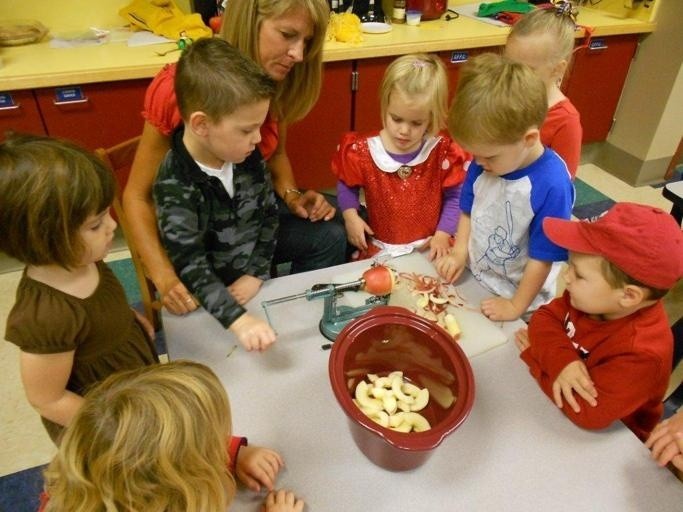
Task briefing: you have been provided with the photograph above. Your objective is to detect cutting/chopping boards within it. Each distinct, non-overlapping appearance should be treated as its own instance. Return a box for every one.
[331,250,509,360]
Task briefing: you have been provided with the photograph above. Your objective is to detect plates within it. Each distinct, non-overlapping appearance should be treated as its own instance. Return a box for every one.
[361,22,392,34]
[0,36,36,45]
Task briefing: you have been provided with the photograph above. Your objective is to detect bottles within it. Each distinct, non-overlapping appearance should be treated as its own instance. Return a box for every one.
[392,0,406,24]
[406,10,422,26]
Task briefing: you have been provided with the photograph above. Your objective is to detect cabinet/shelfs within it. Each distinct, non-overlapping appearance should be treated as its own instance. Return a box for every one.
[0,78,160,230]
[354,48,496,141]
[560,34,642,145]
[270,61,350,191]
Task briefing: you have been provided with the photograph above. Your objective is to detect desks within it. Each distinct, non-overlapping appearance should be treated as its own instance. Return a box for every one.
[158,241,681,506]
[664,180,683,224]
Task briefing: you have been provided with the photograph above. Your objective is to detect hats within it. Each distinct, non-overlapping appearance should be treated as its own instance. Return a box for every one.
[542,202,683,290]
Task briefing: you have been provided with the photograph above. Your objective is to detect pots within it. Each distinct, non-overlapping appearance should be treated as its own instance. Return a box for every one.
[329,306,476,473]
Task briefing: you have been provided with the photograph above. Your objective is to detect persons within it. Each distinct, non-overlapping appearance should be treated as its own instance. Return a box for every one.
[116,0,372,320]
[151,32,286,352]
[512,198,683,440]
[22,358,307,512]
[436,50,578,328]
[1,126,165,447]
[500,6,585,182]
[643,406,683,472]
[329,50,468,265]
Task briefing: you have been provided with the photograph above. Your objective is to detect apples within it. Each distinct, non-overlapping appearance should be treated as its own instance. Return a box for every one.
[364,265,395,297]
